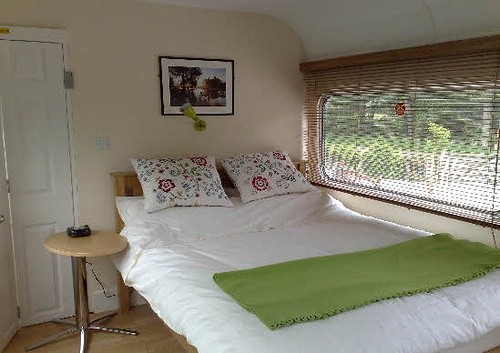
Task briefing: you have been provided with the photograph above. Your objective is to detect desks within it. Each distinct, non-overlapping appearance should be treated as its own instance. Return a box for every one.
[24,229,138,353]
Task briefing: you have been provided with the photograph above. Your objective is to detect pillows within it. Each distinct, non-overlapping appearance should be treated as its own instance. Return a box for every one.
[221,151,314,204]
[130,154,232,214]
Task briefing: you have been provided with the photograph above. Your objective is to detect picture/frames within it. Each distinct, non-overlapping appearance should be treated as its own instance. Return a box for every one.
[157,55,235,117]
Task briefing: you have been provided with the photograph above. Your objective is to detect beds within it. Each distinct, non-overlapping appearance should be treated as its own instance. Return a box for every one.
[110,161,500,353]
